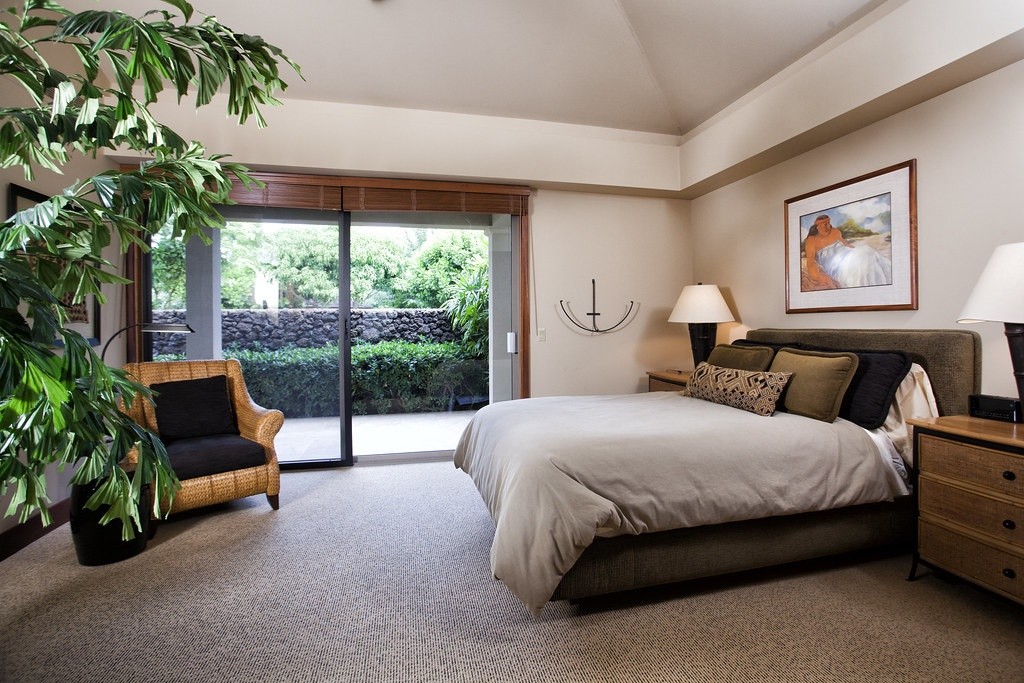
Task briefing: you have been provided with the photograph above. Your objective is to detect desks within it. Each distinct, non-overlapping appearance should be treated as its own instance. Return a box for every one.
[68,462,148,567]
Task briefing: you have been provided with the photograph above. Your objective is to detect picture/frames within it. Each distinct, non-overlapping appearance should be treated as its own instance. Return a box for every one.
[783,158,918,313]
[8,183,102,347]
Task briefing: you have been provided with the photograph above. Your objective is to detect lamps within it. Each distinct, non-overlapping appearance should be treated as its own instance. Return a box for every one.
[667,282,736,368]
[101,322,196,362]
[956,242,1024,419]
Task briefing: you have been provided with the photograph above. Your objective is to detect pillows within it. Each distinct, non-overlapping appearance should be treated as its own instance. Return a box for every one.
[730,339,912,430]
[706,343,772,372]
[767,348,858,424]
[681,360,793,417]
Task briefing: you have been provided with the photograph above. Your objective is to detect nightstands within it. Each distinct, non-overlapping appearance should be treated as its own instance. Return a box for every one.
[905,416,1024,605]
[646,369,693,393]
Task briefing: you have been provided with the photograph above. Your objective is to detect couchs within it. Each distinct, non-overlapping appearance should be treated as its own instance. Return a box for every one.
[119,358,285,540]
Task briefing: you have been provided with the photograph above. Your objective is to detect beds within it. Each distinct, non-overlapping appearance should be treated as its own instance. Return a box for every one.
[454,327,981,604]
